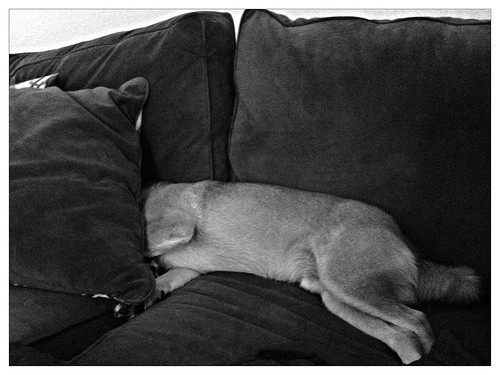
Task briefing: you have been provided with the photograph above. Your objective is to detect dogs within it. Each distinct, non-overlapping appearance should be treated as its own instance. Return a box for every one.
[112,180,480,365]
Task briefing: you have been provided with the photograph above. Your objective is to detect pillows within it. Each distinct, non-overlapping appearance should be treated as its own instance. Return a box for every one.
[10,76,154,306]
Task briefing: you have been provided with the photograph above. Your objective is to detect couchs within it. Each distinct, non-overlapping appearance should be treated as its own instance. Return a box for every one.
[10,8,492,367]
